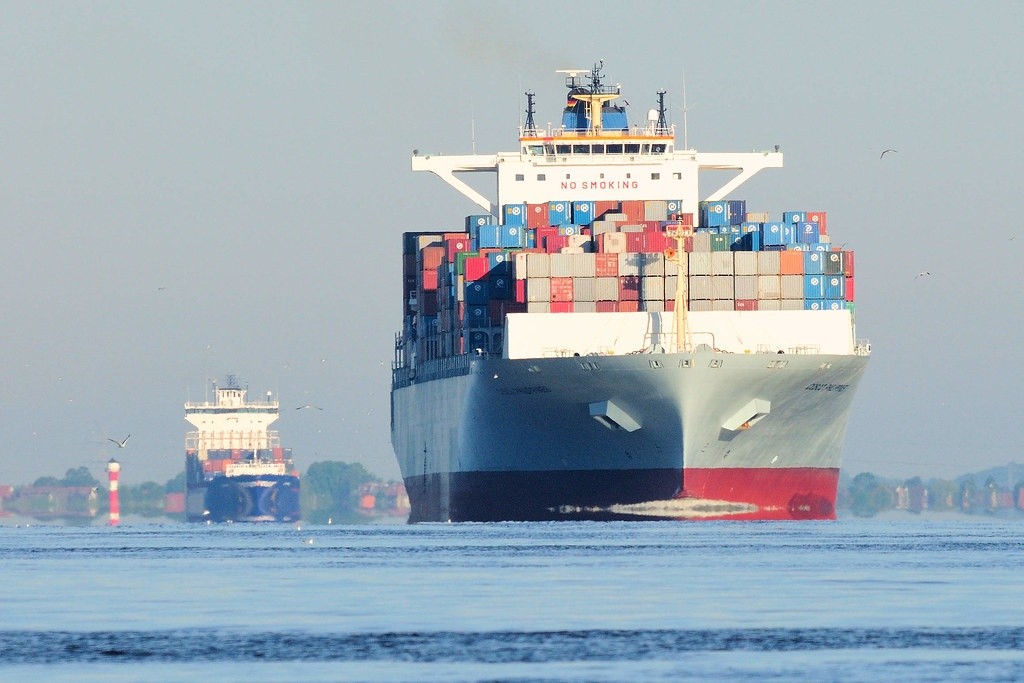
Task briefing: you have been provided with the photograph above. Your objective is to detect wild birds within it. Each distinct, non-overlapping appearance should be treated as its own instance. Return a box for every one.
[107,434,132,449]
[878,149,898,160]
[913,271,932,281]
[296,405,324,411]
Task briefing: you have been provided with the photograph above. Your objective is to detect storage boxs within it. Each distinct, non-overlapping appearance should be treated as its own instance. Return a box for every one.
[400,199,855,363]
[182,447,299,488]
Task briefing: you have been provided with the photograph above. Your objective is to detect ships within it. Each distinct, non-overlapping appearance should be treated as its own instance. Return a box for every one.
[387,61,872,521]
[184,373,303,523]
